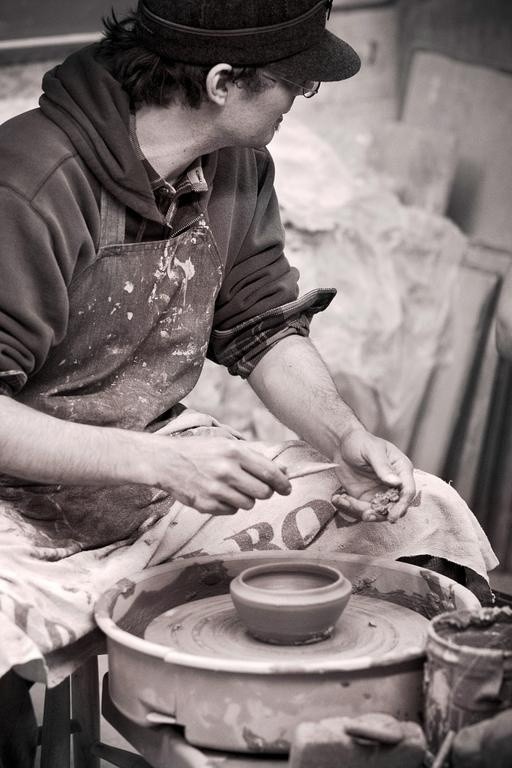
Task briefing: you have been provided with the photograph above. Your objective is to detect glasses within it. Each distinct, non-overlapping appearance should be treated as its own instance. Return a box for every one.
[267,76,320,98]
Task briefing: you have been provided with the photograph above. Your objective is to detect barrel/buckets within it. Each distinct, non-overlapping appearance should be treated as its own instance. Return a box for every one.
[423,606,512,768]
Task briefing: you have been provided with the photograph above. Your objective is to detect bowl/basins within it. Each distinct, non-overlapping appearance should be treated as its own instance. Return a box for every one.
[229,560,355,648]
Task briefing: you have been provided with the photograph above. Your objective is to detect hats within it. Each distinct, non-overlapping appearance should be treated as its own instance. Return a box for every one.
[136,0,361,81]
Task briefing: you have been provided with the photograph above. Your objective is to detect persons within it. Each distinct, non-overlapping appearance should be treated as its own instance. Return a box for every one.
[0,2,500,683]
